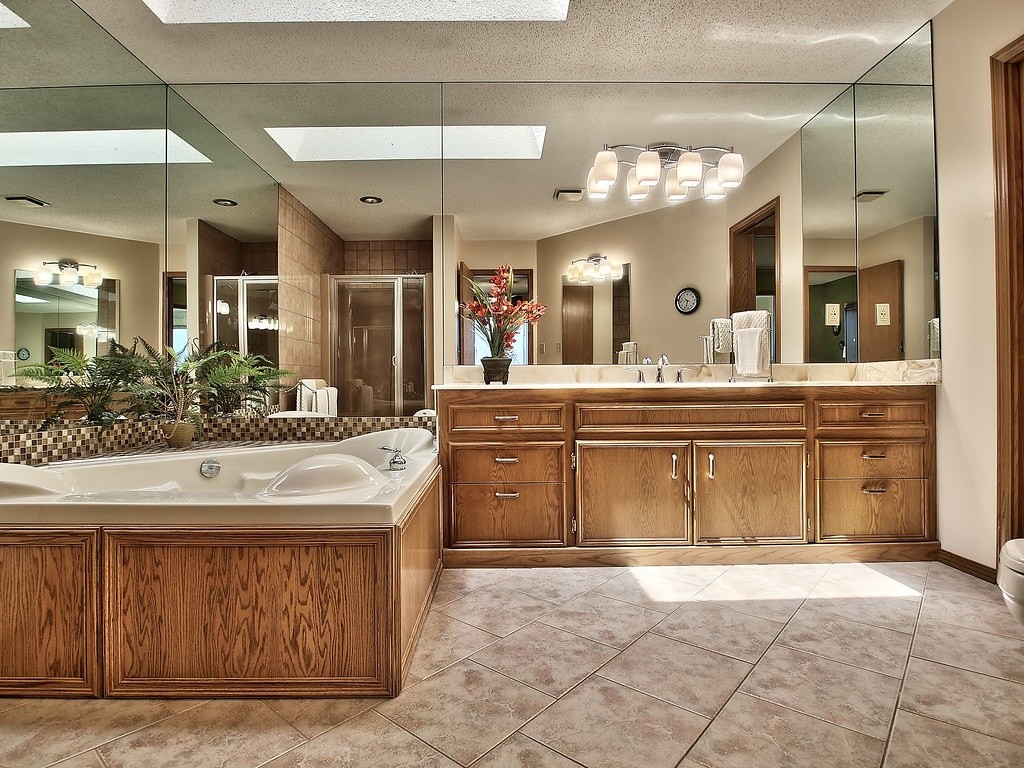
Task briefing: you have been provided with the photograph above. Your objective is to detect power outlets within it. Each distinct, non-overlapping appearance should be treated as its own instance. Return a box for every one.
[875,303,892,327]
[825,303,841,327]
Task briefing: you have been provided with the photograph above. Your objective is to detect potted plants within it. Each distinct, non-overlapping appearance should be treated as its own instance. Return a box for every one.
[9,337,154,432]
[96,338,299,449]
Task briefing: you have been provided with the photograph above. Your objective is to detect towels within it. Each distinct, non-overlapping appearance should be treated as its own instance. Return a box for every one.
[728,327,776,380]
[617,341,638,365]
[702,309,779,364]
[927,317,940,359]
[345,377,375,417]
[295,377,340,418]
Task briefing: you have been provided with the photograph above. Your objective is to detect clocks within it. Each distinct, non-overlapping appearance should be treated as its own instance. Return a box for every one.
[675,287,701,315]
[17,348,30,360]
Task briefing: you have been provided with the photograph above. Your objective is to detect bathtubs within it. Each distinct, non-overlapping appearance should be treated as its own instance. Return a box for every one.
[1,427,439,530]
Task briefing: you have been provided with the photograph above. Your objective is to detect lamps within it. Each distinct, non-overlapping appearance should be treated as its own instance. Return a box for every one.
[247,314,278,331]
[587,143,743,202]
[33,260,103,288]
[76,323,99,338]
[216,299,230,315]
[567,255,623,284]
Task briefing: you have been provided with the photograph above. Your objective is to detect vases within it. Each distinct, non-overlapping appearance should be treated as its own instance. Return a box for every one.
[480,356,512,384]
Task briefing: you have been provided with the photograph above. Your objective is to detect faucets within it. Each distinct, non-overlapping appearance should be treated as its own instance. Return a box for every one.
[653,356,667,383]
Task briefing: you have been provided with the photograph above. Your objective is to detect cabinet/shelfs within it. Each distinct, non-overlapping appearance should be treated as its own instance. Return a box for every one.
[812,386,937,543]
[436,390,570,548]
[571,390,810,545]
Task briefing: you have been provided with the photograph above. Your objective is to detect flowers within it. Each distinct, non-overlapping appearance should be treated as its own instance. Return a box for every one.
[457,264,548,385]
